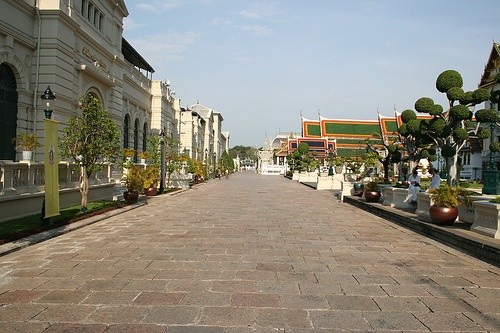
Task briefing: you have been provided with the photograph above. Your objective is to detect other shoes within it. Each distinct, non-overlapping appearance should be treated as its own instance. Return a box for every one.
[409,200,417,204]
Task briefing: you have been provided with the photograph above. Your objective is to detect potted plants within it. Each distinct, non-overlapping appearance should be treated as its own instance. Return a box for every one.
[364,181,382,202]
[429,185,472,224]
[194,161,206,183]
[120,149,158,202]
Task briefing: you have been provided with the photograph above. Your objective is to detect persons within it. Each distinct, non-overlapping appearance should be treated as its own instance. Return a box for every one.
[426,168,441,193]
[225,168,229,180]
[217,168,221,179]
[408,170,420,204]
